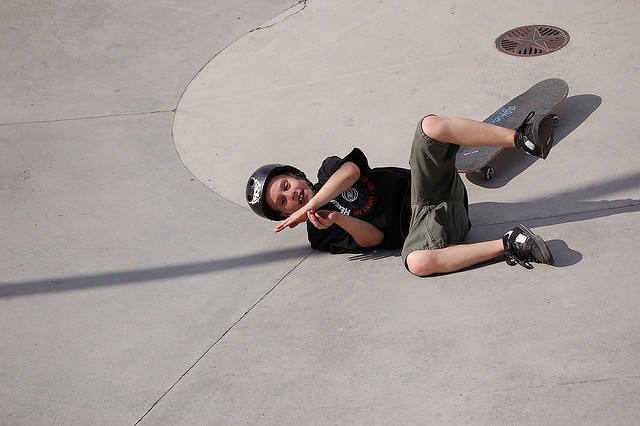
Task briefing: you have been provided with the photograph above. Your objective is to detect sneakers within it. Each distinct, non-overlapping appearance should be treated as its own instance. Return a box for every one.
[503,223,554,269]
[515,111,554,159]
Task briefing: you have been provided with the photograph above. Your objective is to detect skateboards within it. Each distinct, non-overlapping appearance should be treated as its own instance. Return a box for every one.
[455,78,569,181]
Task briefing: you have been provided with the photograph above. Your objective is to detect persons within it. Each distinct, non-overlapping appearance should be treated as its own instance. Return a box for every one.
[245,114,558,278]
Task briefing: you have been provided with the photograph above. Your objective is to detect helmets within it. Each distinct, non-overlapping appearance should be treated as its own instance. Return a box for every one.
[246,164,306,221]
